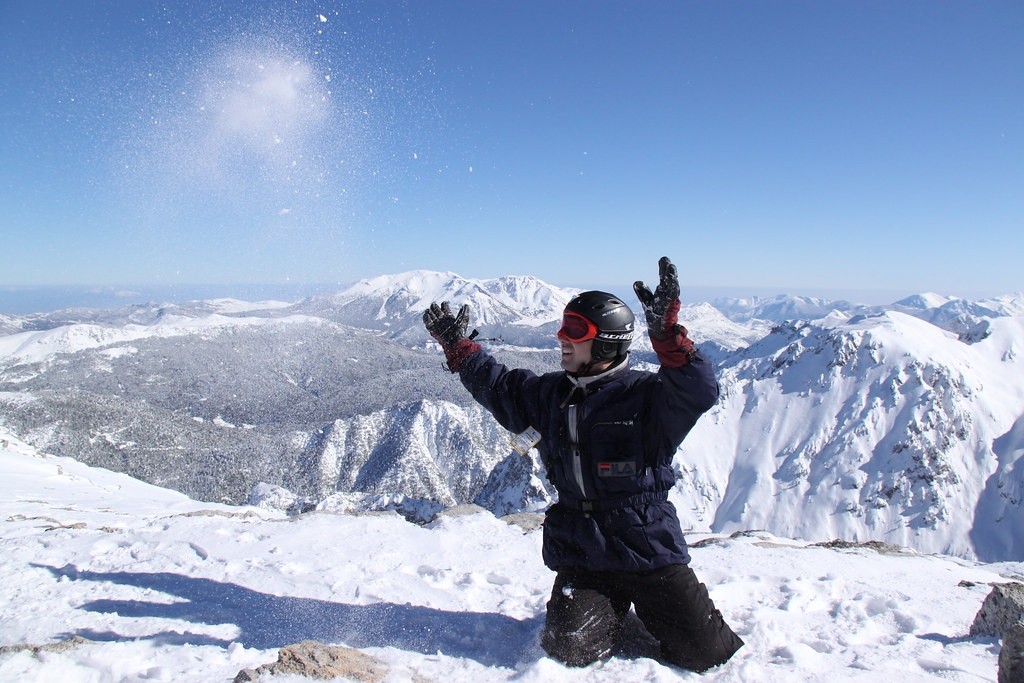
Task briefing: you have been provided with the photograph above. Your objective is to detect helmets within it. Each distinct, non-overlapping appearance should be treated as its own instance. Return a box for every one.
[564,290,635,377]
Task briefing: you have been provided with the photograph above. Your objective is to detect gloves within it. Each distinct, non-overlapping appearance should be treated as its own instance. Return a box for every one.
[633,256,699,368]
[423,300,482,374]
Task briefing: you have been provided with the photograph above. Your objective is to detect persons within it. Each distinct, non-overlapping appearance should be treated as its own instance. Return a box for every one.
[423,257,745,673]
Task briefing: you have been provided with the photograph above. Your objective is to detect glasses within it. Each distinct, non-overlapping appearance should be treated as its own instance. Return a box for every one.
[557,311,598,343]
